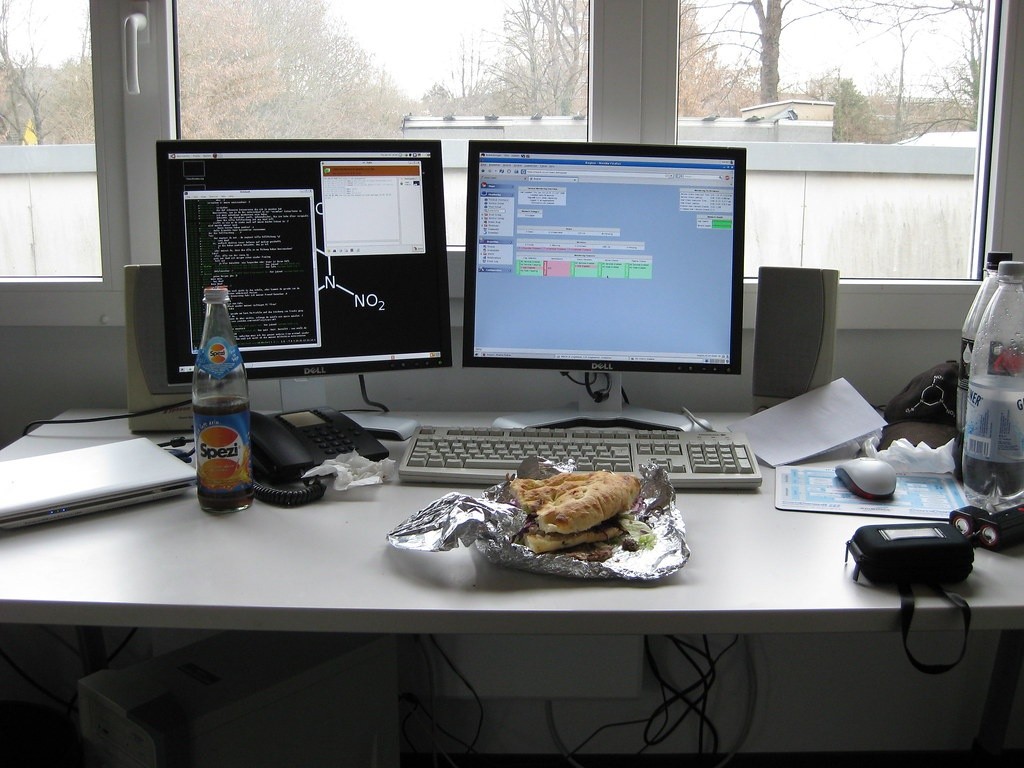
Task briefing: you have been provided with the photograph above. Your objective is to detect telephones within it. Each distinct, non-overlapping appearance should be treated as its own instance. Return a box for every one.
[248,406,391,482]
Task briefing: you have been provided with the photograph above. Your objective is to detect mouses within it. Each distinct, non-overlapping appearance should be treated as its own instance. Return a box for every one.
[835,456,897,498]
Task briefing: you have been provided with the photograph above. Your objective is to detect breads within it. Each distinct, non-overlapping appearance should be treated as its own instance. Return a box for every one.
[509,470,641,536]
[519,513,635,554]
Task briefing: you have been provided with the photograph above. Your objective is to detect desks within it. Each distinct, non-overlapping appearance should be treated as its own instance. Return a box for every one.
[0,405,1024,768]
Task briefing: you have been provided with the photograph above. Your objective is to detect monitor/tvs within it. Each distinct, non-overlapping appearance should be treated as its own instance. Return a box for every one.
[154,138,452,415]
[461,140,748,431]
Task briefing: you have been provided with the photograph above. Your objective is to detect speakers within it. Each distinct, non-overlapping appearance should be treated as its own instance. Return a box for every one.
[749,266,839,418]
[125,264,194,433]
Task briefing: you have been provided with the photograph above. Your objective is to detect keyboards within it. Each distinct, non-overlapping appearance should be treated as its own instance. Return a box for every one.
[398,426,761,491]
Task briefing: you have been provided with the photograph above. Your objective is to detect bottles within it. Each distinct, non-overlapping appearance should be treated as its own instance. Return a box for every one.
[962,261,1024,514]
[191,286,255,513]
[951,251,1014,482]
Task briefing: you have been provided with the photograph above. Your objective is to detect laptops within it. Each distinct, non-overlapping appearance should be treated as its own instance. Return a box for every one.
[0,437,198,531]
